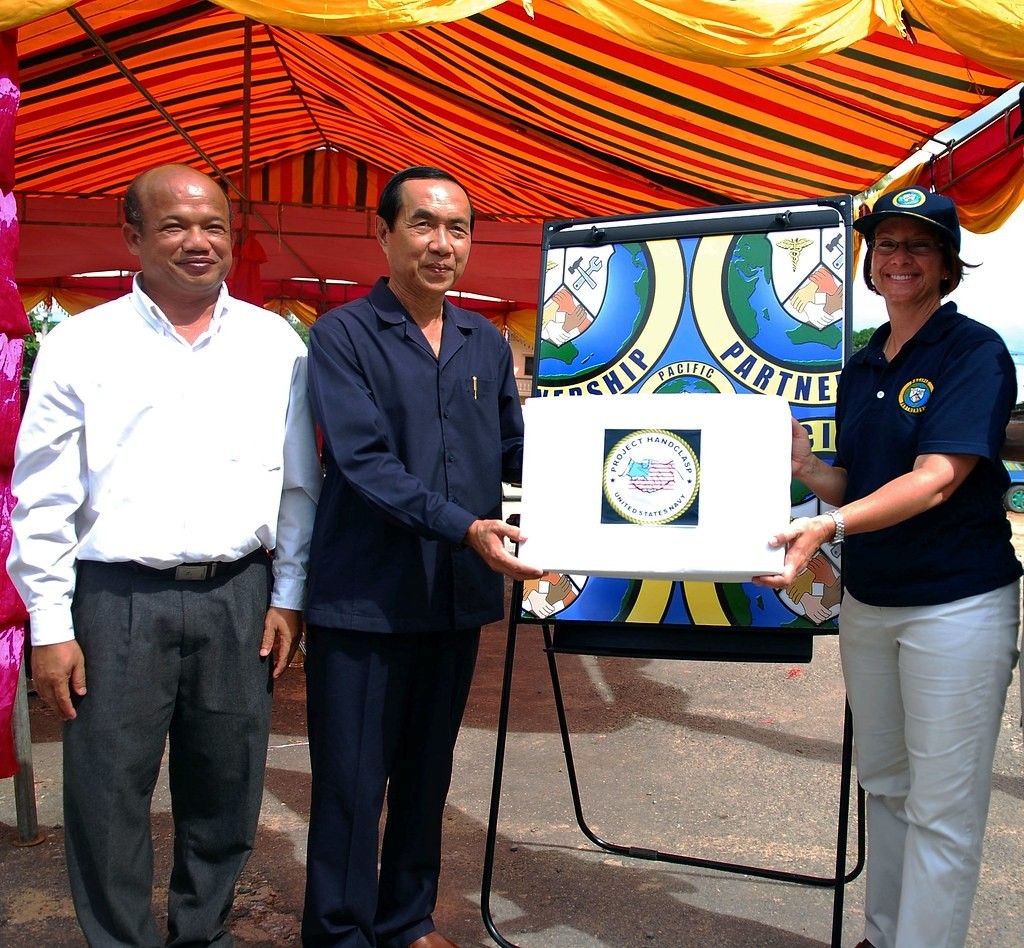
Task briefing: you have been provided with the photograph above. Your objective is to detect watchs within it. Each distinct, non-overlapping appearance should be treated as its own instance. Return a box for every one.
[823,508,845,546]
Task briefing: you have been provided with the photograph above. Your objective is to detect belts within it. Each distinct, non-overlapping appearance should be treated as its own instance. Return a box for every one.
[125,546,263,585]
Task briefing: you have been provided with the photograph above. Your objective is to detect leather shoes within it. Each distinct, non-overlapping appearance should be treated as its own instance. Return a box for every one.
[415,930,462,948]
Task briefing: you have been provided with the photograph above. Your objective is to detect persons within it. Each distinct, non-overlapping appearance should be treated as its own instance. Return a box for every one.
[750,186,1024,947]
[5,162,325,948]
[302,167,550,947]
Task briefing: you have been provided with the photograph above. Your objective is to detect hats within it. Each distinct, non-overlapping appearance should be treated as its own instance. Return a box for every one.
[854,189,963,241]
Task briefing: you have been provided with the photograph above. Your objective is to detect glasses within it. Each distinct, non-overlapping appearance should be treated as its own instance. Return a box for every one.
[866,236,942,256]
